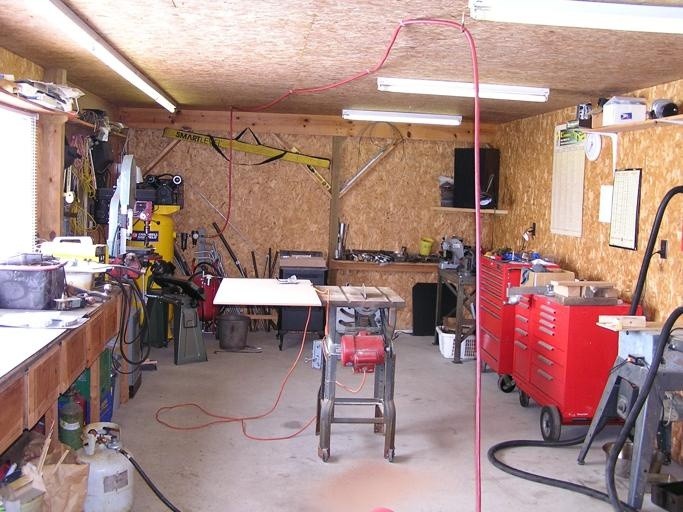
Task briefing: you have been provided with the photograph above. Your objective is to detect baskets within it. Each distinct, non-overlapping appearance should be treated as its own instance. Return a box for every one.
[435,326,476,359]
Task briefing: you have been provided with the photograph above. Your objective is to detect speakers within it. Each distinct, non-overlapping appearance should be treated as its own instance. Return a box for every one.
[412,282,458,336]
[453,147,500,209]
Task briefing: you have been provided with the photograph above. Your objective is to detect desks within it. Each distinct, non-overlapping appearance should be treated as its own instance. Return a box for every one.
[1,284,130,458]
[330,254,476,364]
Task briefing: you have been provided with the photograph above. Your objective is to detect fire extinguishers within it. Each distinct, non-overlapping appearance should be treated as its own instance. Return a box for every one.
[60,385,87,451]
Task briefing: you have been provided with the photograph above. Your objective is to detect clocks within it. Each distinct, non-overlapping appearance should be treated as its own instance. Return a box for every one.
[585,132,601,161]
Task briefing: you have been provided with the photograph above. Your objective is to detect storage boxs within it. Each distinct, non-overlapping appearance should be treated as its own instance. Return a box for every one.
[591,96,646,126]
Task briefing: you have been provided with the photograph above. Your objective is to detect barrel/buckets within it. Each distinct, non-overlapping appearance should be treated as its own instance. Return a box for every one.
[216,314,249,352]
